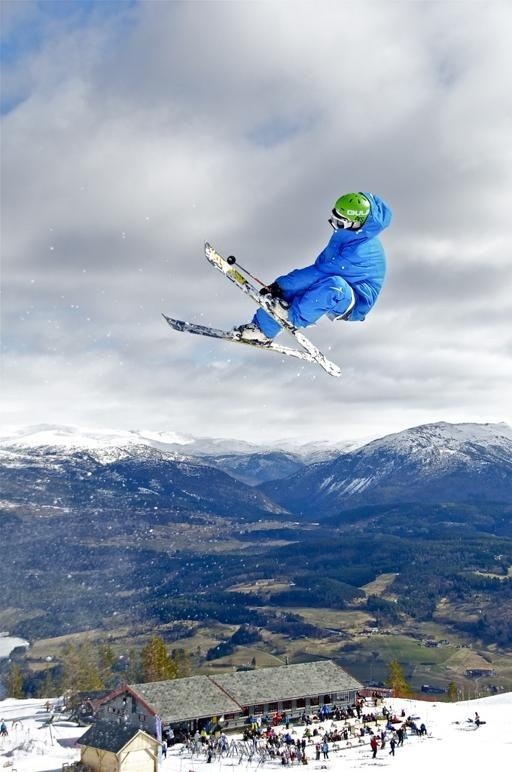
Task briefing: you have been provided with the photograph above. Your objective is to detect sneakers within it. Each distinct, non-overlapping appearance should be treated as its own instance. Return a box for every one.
[231,323,273,344]
[263,293,297,330]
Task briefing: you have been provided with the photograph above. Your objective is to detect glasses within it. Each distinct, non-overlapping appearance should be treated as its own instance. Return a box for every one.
[331,208,353,230]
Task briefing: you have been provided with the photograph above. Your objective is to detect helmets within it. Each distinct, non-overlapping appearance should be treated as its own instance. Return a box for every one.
[328,192,370,231]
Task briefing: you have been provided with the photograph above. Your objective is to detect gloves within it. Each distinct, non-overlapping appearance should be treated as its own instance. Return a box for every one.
[259,282,282,298]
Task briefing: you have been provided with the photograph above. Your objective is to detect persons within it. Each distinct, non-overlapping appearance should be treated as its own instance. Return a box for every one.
[159,686,427,765]
[474,711,480,727]
[0,721,9,735]
[235,192,394,342]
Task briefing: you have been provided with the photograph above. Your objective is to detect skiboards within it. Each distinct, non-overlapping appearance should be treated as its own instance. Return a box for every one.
[160,242,342,379]
